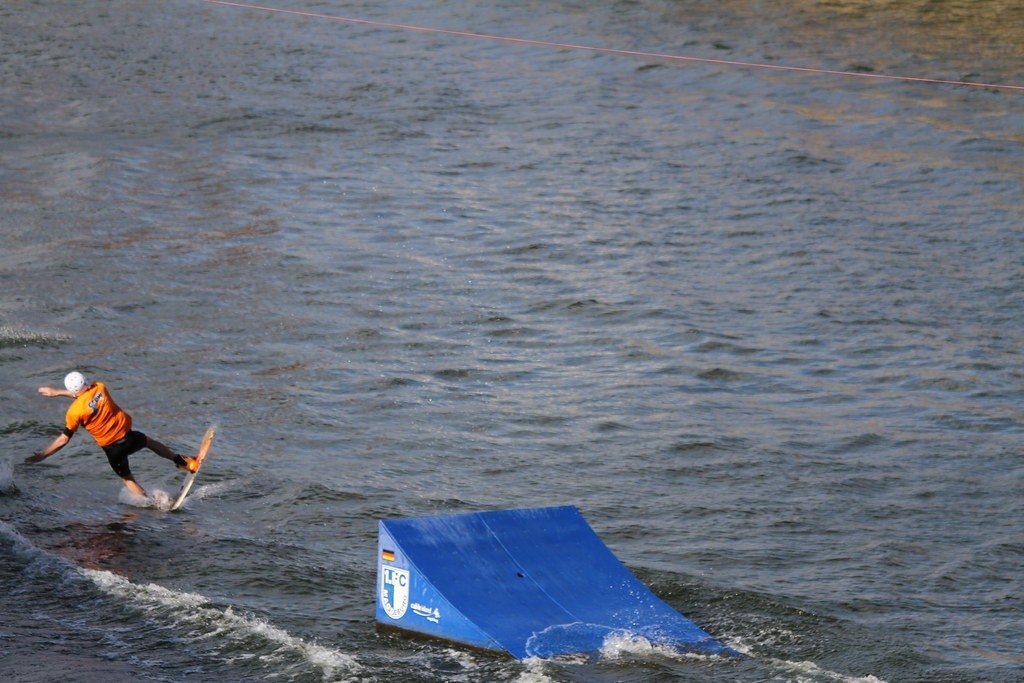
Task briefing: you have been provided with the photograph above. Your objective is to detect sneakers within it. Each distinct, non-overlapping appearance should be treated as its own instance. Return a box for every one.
[175,454,198,473]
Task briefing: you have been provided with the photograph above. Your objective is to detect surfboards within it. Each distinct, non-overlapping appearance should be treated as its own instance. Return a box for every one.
[170,427,214,511]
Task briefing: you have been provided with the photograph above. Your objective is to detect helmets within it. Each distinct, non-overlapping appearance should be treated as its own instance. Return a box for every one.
[64,371,85,393]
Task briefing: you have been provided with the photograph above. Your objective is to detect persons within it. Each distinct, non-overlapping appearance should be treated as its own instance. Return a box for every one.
[24,372,199,496]
